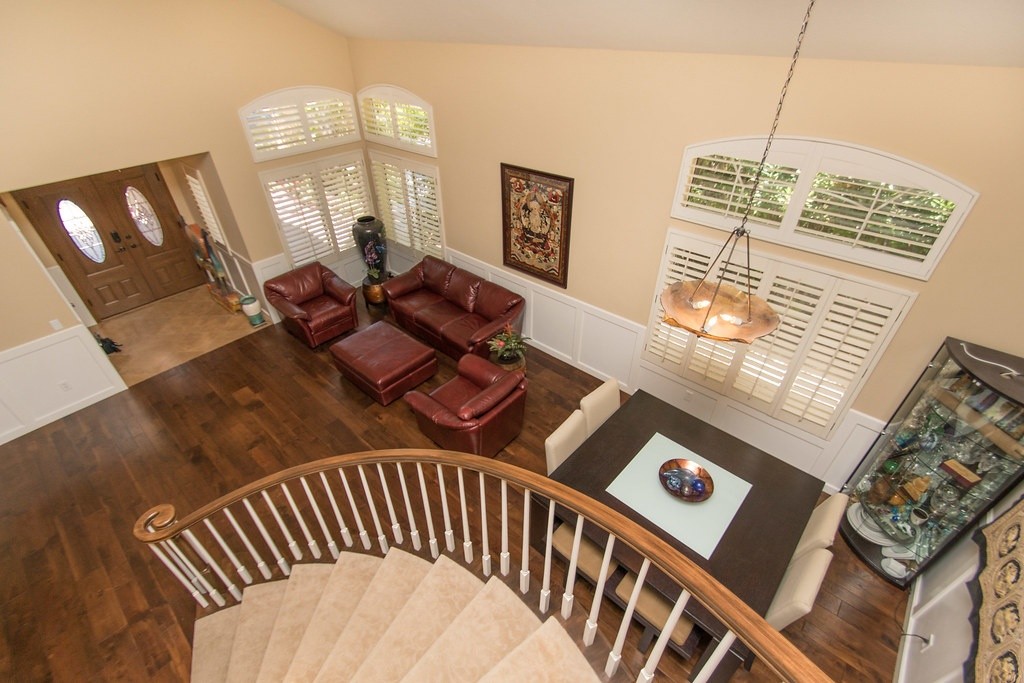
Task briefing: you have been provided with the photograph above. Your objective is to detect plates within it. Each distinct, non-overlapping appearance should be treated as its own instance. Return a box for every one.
[659,459,715,503]
[846,502,899,546]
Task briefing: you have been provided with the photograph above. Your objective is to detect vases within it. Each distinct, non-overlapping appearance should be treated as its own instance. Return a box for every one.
[368,270,381,283]
[490,352,528,378]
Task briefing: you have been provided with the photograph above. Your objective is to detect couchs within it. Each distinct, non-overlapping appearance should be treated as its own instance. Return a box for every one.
[264,261,357,353]
[381,256,525,362]
[402,353,529,472]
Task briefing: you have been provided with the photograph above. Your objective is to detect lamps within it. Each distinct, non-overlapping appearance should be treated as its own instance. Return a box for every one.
[659,0,817,343]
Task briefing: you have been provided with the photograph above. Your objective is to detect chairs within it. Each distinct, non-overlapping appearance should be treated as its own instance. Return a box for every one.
[543,378,848,670]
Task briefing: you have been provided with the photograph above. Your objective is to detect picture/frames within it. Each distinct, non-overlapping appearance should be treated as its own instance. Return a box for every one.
[500,163,575,289]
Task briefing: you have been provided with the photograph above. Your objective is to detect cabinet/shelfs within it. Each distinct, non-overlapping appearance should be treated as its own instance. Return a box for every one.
[842,340,1023,590]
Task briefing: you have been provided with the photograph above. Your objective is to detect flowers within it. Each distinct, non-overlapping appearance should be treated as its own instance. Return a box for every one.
[486,323,531,360]
[364,243,378,271]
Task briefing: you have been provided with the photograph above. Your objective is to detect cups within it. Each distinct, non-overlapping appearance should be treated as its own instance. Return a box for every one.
[911,507,929,526]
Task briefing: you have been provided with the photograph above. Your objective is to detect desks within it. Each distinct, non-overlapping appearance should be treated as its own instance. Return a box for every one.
[527,390,825,683]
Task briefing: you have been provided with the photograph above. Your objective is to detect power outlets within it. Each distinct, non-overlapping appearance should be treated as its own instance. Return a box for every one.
[919,634,934,653]
